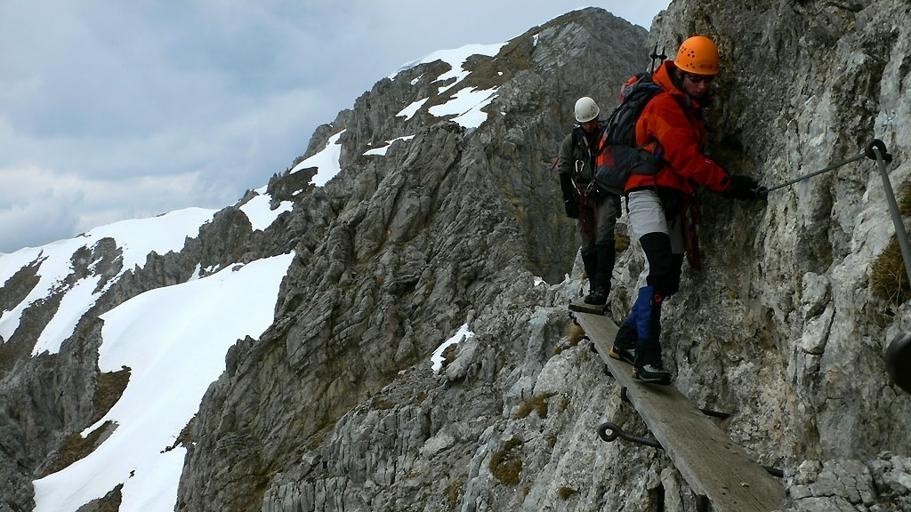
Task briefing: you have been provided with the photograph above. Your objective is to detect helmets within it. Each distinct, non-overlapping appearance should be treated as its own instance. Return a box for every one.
[674,36,720,76]
[574,97,600,123]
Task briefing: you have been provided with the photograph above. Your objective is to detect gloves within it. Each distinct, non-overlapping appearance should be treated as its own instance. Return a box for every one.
[730,175,756,200]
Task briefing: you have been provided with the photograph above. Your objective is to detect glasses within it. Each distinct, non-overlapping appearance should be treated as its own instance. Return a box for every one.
[686,73,715,83]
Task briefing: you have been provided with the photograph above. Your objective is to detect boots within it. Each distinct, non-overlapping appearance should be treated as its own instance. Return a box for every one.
[582,246,615,305]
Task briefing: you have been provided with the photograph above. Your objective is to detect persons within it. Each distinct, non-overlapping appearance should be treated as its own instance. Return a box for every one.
[557,97,622,305]
[608,37,759,387]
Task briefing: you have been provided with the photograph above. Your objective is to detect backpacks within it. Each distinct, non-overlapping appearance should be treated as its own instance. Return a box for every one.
[594,73,663,195]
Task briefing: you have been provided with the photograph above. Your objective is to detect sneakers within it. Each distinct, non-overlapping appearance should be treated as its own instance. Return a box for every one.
[609,341,672,386]
